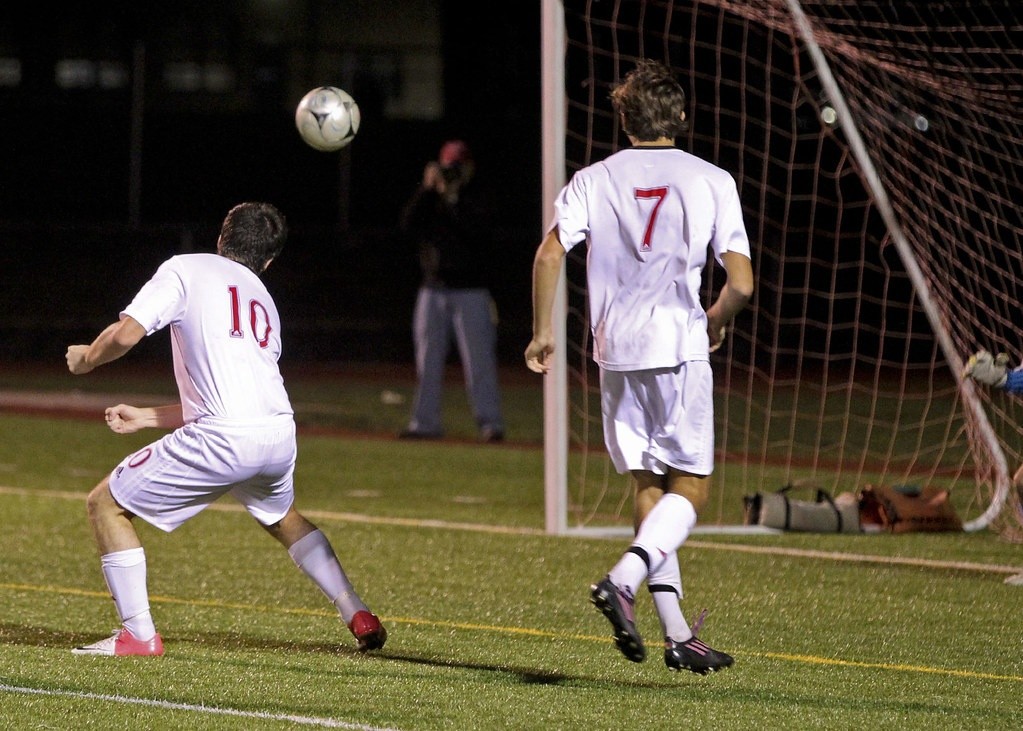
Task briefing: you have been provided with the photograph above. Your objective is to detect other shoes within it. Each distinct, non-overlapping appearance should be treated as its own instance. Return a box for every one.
[482,427,507,445]
[397,424,439,442]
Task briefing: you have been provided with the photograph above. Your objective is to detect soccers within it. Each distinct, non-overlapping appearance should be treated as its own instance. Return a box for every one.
[295,84,362,151]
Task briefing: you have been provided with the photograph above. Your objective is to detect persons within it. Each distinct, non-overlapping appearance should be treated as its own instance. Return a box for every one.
[65,200,387,656]
[526,60,754,675]
[962,347,1023,393]
[397,142,507,443]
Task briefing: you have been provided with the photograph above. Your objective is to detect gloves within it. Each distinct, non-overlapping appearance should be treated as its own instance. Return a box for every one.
[961,349,1010,386]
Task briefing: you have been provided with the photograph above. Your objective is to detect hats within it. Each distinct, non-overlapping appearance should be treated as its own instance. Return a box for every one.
[439,140,475,166]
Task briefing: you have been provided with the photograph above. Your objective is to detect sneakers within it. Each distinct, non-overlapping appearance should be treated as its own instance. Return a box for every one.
[587,577,648,664]
[664,608,735,676]
[347,610,388,651]
[71,626,163,656]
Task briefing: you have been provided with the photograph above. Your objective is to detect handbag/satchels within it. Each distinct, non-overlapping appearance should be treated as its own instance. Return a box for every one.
[744,479,861,533]
[854,482,966,534]
